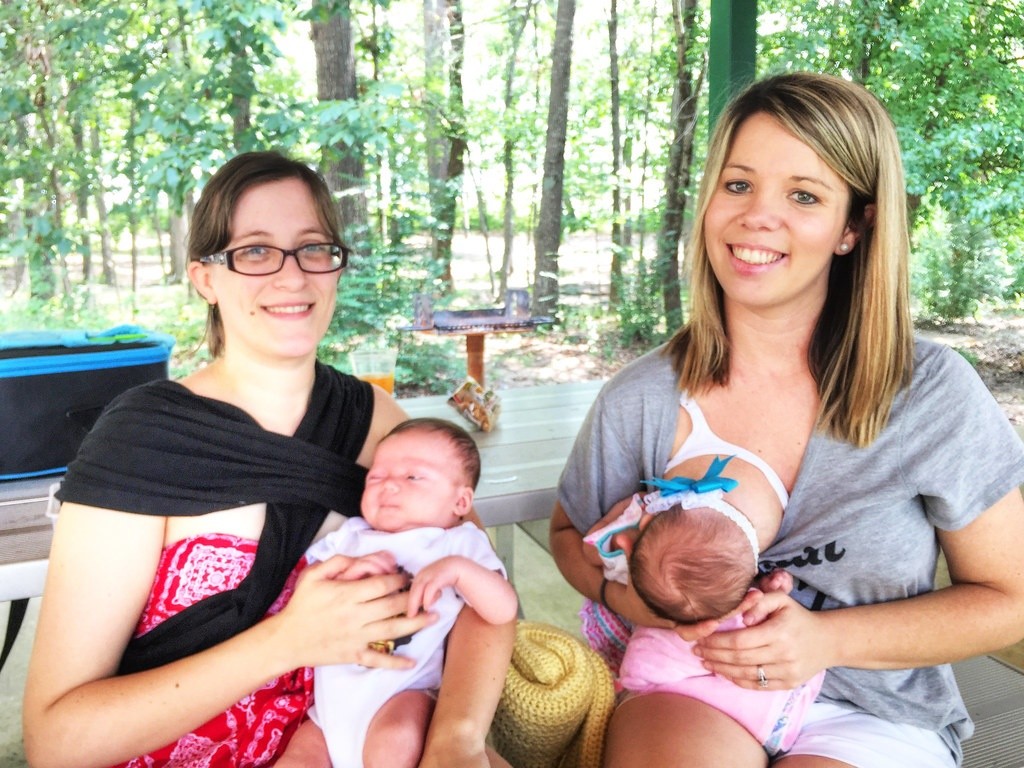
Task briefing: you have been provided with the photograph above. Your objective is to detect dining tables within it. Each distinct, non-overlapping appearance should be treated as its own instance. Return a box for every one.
[0,383,607,603]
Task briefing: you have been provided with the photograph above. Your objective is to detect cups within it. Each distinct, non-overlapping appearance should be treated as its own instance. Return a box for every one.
[348,345,398,399]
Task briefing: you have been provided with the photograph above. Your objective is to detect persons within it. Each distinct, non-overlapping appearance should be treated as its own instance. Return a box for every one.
[549,76,1024,768]
[272,417,519,768]
[23,154,514,768]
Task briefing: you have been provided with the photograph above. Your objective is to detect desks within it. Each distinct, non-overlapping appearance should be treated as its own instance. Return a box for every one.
[417,312,563,391]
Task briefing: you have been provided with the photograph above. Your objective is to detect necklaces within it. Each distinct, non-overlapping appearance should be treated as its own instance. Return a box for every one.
[758,664,768,688]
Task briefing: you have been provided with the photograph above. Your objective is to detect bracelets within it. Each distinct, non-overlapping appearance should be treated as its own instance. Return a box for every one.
[600,578,618,615]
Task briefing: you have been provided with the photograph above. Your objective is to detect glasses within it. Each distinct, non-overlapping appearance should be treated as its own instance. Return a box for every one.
[196,243,348,276]
